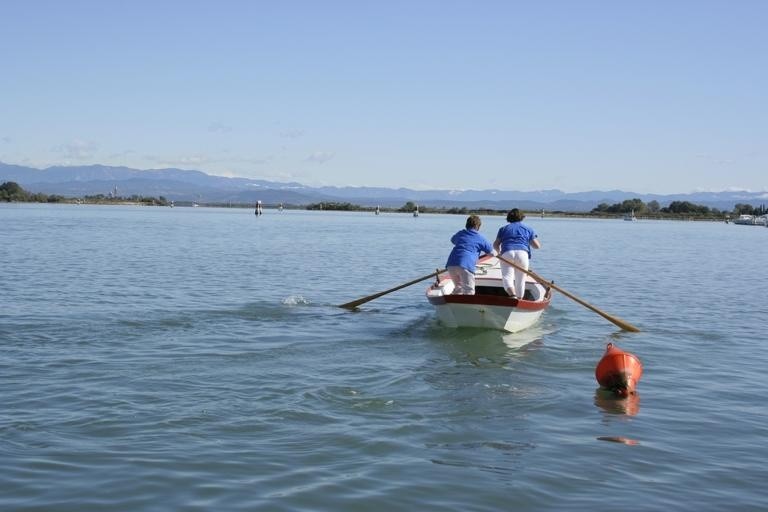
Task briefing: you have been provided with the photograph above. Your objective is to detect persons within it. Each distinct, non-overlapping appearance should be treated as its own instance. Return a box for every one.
[445,215,498,295]
[492,208,540,300]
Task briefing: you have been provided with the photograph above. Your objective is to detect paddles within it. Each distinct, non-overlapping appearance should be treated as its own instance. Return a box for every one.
[339,253,489,309]
[497,252,643,331]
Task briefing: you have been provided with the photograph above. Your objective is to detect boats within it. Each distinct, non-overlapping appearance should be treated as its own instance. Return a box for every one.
[427,246,553,332]
